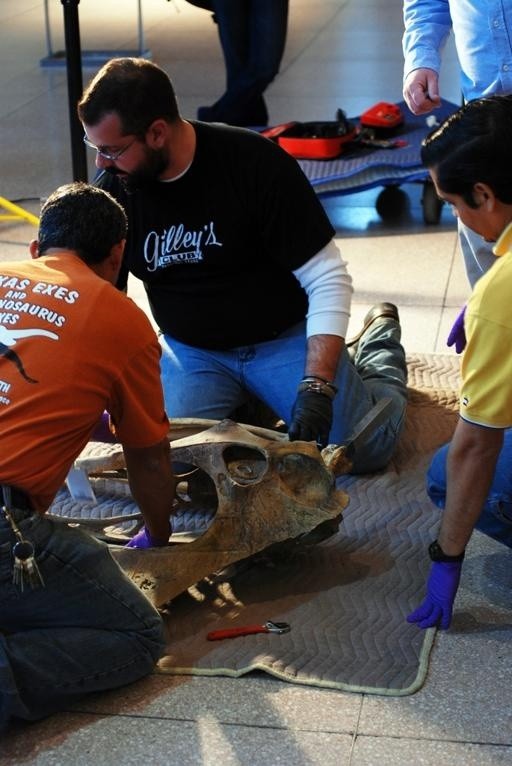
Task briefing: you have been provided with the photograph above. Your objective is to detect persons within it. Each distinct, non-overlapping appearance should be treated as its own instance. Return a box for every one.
[179,0,289,129]
[42,56,408,486]
[390,0,512,293]
[2,173,180,742]
[395,94,510,631]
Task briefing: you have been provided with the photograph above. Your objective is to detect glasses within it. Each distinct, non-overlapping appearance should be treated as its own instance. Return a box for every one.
[81,132,136,163]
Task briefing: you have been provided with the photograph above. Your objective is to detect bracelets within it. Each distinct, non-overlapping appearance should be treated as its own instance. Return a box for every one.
[297,376,338,399]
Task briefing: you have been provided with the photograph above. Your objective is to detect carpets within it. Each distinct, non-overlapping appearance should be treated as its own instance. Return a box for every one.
[40,354,467,696]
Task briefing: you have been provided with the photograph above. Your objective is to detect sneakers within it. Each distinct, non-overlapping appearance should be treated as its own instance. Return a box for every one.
[196,93,271,126]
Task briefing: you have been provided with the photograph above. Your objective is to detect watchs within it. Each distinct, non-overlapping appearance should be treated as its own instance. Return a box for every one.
[428,540,466,562]
